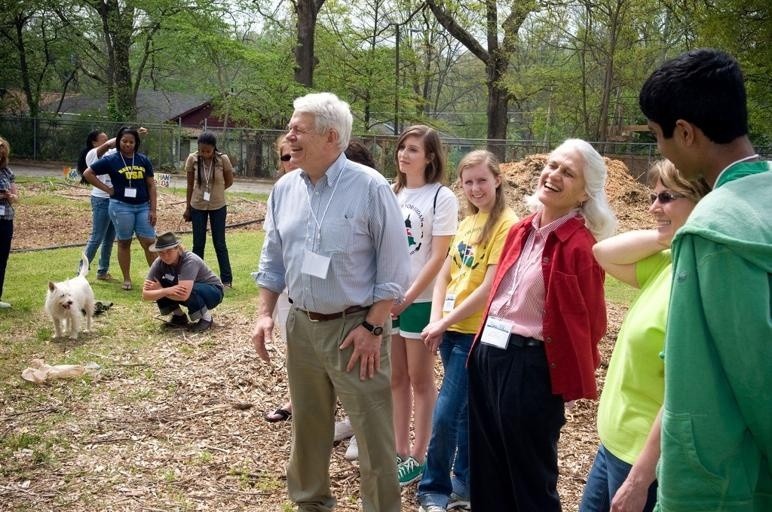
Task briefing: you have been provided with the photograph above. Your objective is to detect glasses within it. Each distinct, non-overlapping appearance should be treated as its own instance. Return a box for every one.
[651,191,686,202]
[281,155,291,161]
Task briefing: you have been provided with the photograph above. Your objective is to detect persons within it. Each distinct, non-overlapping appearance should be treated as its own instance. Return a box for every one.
[142,232,224,334]
[0,137,18,309]
[414,149,522,512]
[184,132,233,288]
[465,138,620,511]
[577,157,710,511]
[641,49,772,510]
[83,126,158,289]
[77,126,149,285]
[389,126,458,487]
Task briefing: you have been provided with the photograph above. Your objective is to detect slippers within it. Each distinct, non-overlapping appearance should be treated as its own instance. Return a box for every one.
[122,283,132,290]
[266,405,291,420]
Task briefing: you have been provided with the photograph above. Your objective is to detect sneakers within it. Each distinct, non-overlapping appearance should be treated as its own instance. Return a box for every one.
[333,419,359,459]
[396,454,470,512]
[98,273,113,280]
[0,302,11,308]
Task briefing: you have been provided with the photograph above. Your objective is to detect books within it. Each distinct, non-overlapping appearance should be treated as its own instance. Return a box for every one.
[251,92,410,512]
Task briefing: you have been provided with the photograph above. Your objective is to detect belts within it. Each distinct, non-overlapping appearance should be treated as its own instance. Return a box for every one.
[305,306,369,322]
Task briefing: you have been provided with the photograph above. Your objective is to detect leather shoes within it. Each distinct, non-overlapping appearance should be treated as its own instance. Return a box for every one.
[166,313,212,331]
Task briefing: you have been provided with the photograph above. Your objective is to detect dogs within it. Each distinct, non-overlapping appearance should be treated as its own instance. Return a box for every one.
[45,252,96,340]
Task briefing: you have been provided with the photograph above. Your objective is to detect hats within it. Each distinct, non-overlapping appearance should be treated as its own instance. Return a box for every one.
[149,232,181,252]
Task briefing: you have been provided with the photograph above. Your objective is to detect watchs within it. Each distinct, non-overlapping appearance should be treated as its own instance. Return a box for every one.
[362,319,384,337]
